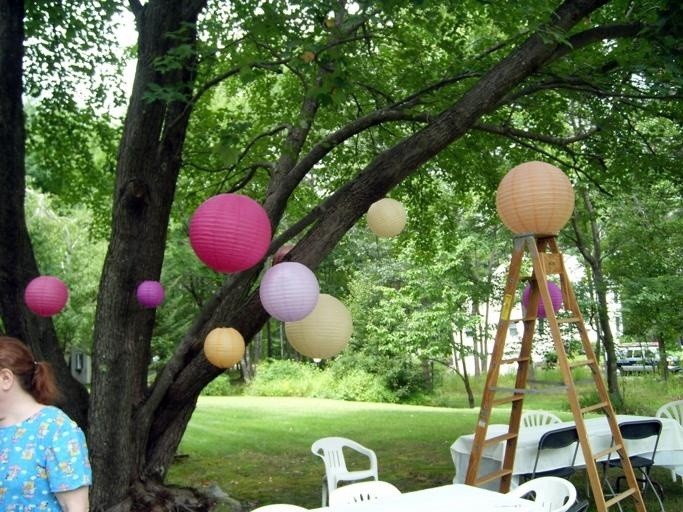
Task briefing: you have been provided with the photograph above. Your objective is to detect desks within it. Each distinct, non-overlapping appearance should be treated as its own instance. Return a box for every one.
[450,414,683,501]
[309,484,547,512]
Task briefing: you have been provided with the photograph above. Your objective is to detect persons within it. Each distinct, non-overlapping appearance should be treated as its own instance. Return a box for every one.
[0,334,92,512]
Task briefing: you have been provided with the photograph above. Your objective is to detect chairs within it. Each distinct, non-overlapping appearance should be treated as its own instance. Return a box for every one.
[520,410,561,427]
[251,503,308,512]
[311,437,402,507]
[498,476,577,512]
[522,425,581,503]
[328,480,402,507]
[601,420,665,500]
[641,400,683,489]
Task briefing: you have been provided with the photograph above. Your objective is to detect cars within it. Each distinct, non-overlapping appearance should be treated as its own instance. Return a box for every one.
[616,346,679,377]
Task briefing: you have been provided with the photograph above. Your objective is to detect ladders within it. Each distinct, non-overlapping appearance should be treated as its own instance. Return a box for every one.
[464,233,646,512]
